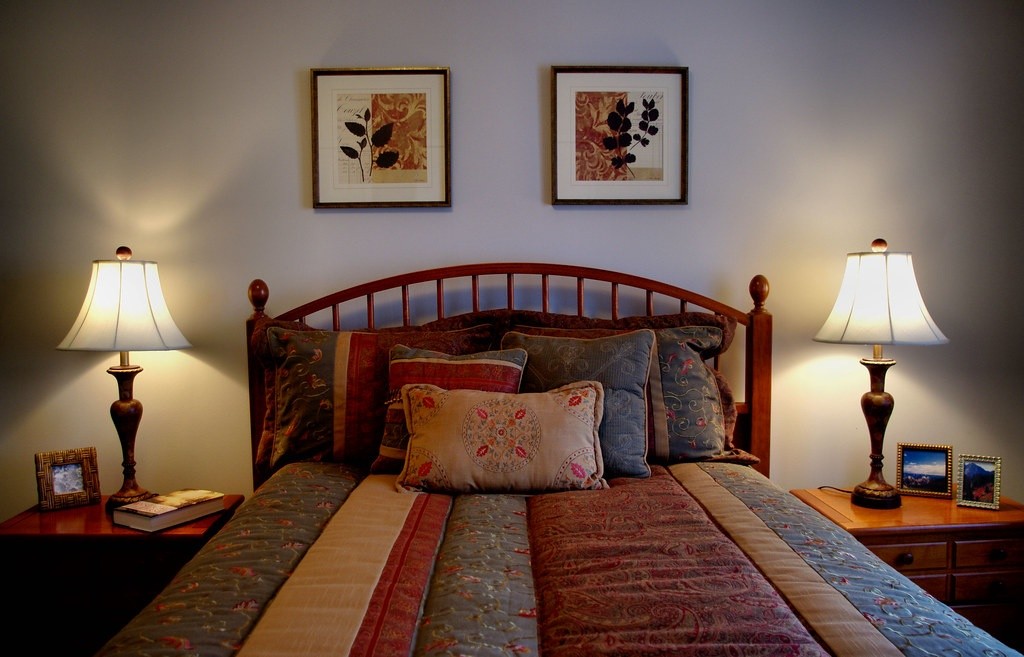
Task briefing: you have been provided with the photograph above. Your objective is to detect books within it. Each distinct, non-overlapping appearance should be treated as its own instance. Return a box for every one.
[113,488,225,532]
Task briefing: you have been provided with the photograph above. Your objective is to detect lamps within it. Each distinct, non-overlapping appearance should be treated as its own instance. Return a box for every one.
[811,236,950,508]
[56,246,192,511]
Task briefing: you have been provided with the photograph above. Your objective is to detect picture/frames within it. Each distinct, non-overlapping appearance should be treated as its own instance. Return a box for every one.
[310,65,454,207]
[549,63,690,204]
[896,442,951,499]
[35,446,101,510]
[957,454,1001,510]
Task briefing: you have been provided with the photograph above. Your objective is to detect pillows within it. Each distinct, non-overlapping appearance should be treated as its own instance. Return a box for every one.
[395,380,608,492]
[501,329,654,479]
[370,345,529,471]
[263,319,501,467]
[517,313,762,466]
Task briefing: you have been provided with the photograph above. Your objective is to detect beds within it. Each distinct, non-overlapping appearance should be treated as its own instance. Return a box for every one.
[89,262,1024,657]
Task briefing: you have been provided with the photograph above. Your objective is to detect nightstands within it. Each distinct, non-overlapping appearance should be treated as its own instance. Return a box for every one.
[791,480,1023,652]
[0,493,245,656]
